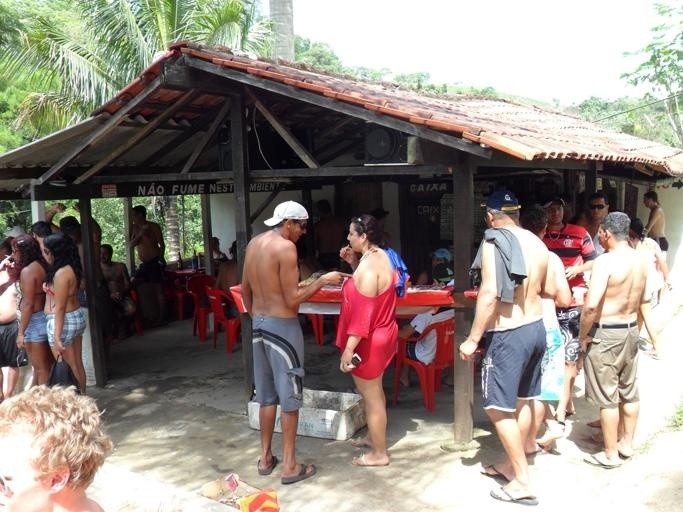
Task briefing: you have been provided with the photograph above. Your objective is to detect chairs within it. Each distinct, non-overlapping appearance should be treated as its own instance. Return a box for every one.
[130,268,240,353]
[303,314,485,413]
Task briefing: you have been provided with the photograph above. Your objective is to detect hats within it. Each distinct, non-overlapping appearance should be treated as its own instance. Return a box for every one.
[541,197,565,208]
[432,263,454,282]
[430,249,451,261]
[480,189,522,210]
[264,201,310,227]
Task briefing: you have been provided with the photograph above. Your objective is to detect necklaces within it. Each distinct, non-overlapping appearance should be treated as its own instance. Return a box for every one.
[545,223,563,241]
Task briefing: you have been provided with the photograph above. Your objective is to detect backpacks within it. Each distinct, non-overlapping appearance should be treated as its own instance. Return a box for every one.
[47,354,80,393]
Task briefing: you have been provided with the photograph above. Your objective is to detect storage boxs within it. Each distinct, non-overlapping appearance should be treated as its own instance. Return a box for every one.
[247,387,366,441]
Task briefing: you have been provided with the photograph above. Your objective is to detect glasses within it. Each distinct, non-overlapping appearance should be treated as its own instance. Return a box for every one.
[591,204,606,209]
[12,249,19,253]
[44,248,50,255]
[357,217,365,233]
[298,221,307,228]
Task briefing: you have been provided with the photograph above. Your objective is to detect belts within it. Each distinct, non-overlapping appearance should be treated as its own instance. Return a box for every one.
[595,322,636,328]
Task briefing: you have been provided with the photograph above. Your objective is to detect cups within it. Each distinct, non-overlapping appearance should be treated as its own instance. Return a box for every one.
[192,256,198,271]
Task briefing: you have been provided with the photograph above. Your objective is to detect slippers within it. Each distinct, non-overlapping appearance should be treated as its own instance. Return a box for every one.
[259,438,390,485]
[481,442,621,507]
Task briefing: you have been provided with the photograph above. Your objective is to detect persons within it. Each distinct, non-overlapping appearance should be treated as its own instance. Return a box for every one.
[628,217,673,361]
[414,270,428,285]
[240,200,352,485]
[518,203,572,454]
[214,240,242,318]
[581,192,607,262]
[578,212,648,470]
[310,198,345,272]
[429,247,452,285]
[458,191,549,506]
[41,232,87,393]
[642,191,673,293]
[99,244,137,339]
[540,193,598,416]
[397,262,455,387]
[372,205,390,233]
[11,235,48,388]
[295,242,325,283]
[127,205,169,329]
[335,212,399,466]
[588,403,624,443]
[557,193,577,224]
[0,236,28,403]
[31,220,52,256]
[44,202,112,338]
[0,383,115,512]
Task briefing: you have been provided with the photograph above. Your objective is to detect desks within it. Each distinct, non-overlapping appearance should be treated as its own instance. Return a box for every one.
[229,282,477,416]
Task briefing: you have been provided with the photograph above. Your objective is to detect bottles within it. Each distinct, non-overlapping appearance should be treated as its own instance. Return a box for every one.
[177,252,183,270]
